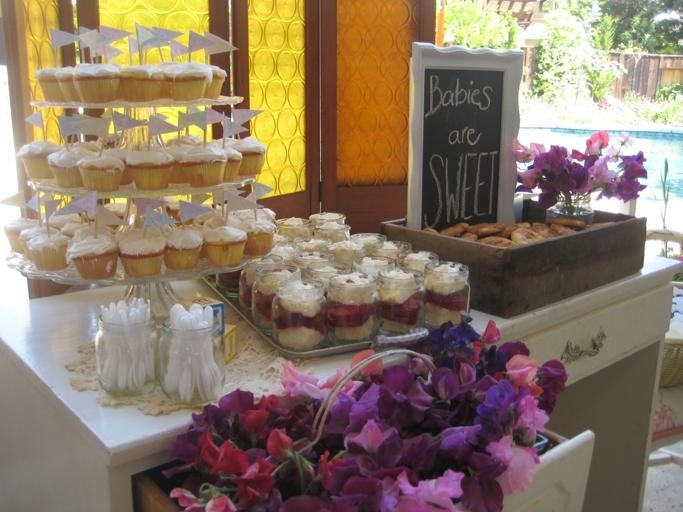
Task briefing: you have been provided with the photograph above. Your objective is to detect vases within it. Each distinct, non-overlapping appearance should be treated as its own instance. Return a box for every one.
[541,191,598,228]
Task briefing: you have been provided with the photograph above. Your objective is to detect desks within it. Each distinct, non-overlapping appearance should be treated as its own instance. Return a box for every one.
[2,235,680,509]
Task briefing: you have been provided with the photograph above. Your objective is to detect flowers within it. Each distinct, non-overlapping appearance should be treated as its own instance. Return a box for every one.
[514,124,655,204]
[164,309,566,509]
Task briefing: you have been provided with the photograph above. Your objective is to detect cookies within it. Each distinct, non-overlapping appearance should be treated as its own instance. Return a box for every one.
[421,218,615,248]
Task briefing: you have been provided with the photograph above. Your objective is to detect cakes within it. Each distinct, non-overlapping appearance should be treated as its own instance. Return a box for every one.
[35,62,227,81]
[4,203,276,259]
[18,135,265,171]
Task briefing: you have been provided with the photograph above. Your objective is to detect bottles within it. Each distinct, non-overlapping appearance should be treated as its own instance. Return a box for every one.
[93,308,159,398]
[205,208,472,357]
[150,313,228,407]
